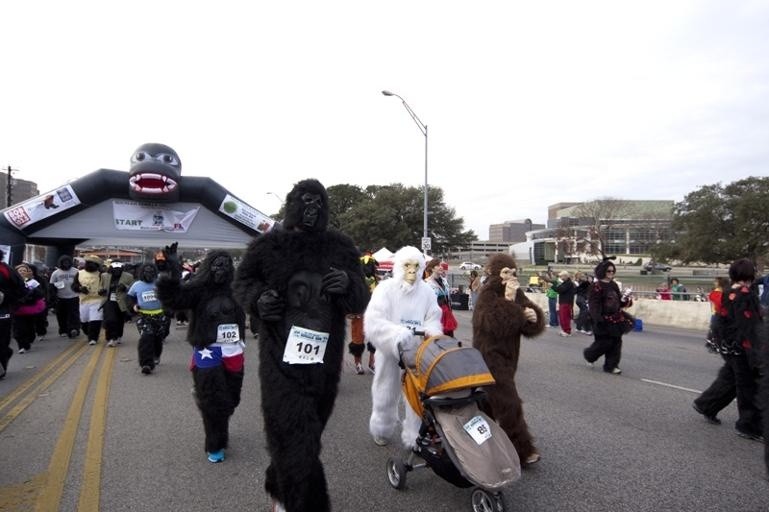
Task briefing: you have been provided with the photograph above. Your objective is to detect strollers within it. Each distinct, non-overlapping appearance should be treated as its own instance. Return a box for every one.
[385,329,522,512]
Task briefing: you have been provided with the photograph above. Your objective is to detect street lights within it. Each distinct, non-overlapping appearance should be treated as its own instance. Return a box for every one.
[265,191,285,208]
[382,90,432,258]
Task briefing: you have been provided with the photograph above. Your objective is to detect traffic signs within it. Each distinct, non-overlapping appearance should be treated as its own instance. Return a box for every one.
[421,237,432,251]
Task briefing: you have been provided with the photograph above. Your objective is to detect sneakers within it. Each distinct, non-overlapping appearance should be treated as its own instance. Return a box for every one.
[154,358,160,365]
[585,360,594,368]
[89,340,96,345]
[38,329,78,341]
[609,367,622,374]
[142,365,152,374]
[107,340,115,347]
[737,429,769,443]
[116,336,122,345]
[367,364,376,374]
[354,362,364,375]
[206,447,226,463]
[526,453,541,465]
[543,322,593,339]
[693,402,721,425]
[18,347,25,353]
[175,320,189,329]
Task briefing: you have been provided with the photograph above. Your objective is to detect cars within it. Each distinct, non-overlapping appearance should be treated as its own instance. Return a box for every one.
[644,260,672,272]
[458,261,480,271]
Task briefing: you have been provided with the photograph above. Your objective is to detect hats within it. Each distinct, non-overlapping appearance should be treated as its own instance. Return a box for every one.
[558,271,569,276]
[72,254,124,269]
[426,257,442,272]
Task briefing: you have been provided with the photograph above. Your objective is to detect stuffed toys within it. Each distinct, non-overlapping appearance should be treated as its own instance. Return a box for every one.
[0,177,544,512]
